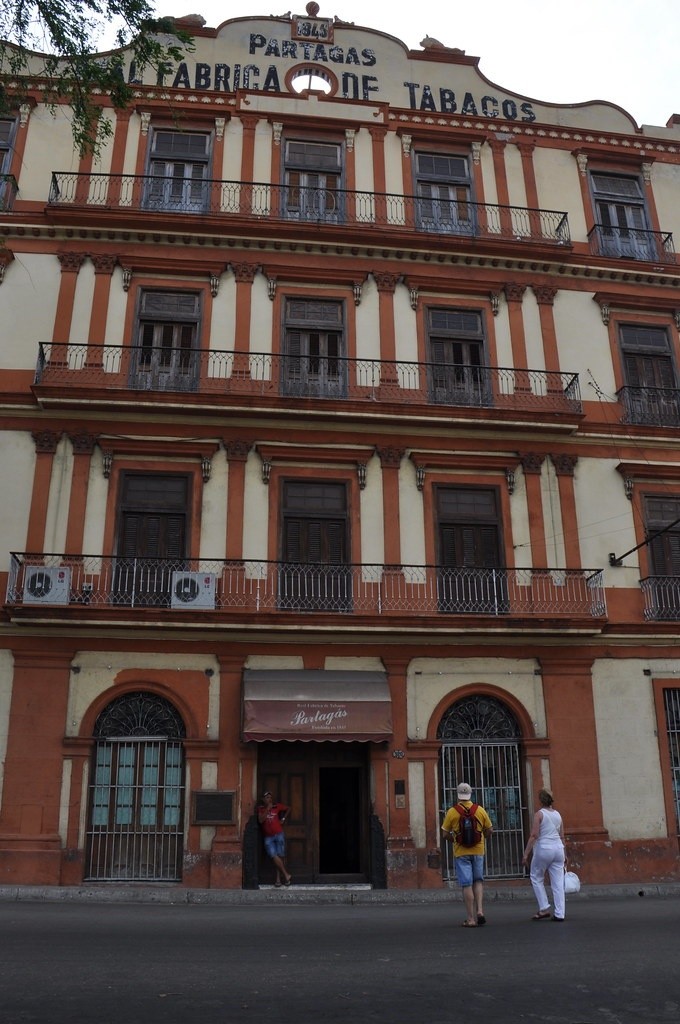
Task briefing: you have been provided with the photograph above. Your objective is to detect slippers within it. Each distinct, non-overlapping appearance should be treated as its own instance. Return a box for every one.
[531,911,550,920]
[477,916,486,926]
[284,874,291,886]
[461,920,478,928]
[548,915,564,922]
[275,880,281,887]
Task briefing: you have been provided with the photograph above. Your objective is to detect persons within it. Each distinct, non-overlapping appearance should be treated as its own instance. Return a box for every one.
[522,787,569,921]
[258,791,292,887]
[441,782,493,927]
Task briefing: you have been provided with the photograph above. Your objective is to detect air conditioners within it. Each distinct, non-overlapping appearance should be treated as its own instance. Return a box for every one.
[171,571,215,609]
[23,566,70,605]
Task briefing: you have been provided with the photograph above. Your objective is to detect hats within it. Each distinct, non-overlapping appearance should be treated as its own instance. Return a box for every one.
[263,791,272,797]
[456,783,472,800]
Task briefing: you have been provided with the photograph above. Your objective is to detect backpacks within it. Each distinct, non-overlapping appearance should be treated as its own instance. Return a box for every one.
[452,804,483,848]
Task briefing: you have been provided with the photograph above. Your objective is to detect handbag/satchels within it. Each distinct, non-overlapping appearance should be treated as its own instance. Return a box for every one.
[564,867,580,893]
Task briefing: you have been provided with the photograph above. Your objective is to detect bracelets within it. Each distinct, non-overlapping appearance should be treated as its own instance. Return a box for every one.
[281,817,286,821]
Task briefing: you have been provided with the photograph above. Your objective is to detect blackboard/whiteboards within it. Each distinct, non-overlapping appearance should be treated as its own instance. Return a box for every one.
[191,789,238,826]
